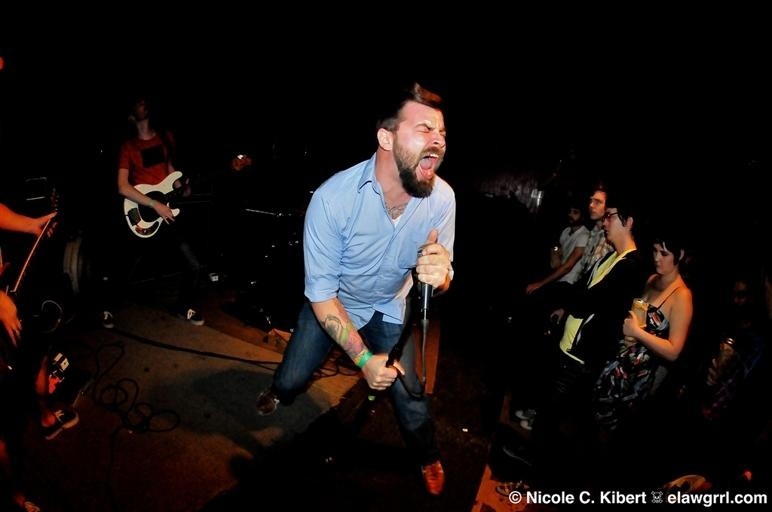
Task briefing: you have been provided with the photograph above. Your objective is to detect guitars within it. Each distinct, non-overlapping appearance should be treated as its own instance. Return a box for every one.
[0,188,62,377]
[124,152,253,238]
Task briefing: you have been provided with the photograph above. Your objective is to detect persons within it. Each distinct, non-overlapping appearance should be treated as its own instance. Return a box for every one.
[115,95,212,326]
[255,77,460,499]
[0,205,81,512]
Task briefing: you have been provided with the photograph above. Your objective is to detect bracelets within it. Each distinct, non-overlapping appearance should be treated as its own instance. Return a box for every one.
[357,352,373,370]
[149,199,156,208]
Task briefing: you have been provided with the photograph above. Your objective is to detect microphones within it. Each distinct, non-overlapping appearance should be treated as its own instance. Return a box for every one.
[417,241,432,333]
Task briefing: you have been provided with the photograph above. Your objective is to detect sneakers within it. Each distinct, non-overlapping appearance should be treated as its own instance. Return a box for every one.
[254,384,281,417]
[515,408,537,430]
[179,308,205,327]
[102,311,116,329]
[420,459,446,495]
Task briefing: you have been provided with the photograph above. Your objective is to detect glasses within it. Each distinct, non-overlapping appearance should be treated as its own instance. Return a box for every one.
[601,212,618,221]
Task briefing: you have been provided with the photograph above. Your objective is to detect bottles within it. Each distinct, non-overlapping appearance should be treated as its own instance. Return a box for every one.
[719,336,734,373]
